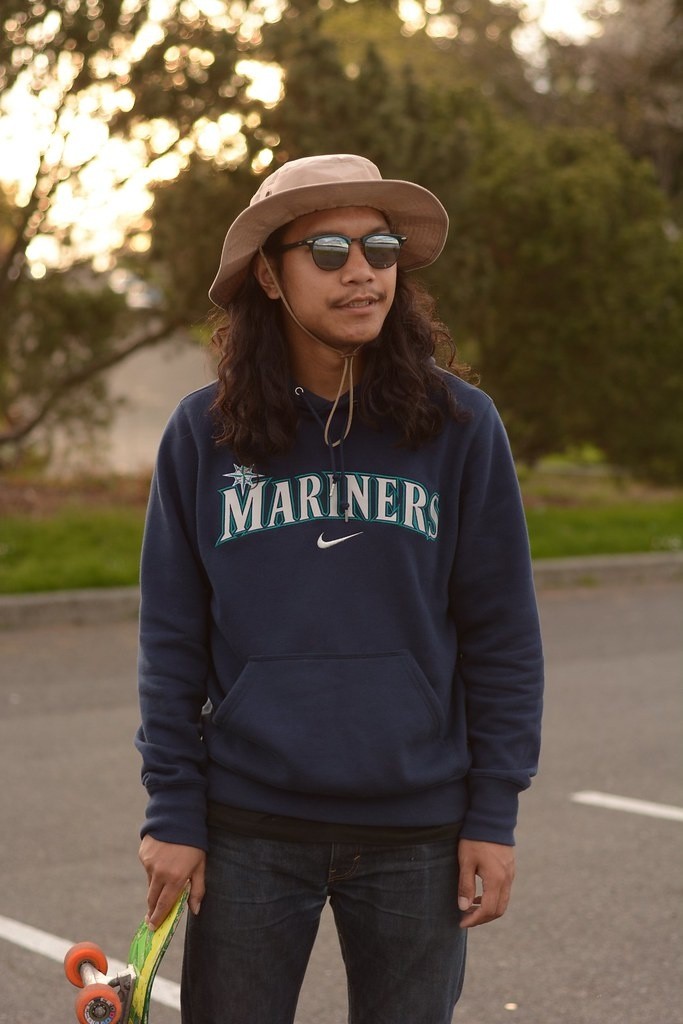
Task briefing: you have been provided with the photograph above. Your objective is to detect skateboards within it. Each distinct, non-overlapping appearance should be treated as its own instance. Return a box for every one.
[63,876,193,1023]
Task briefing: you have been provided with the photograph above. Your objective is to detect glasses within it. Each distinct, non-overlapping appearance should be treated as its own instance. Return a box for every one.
[269,233,408,272]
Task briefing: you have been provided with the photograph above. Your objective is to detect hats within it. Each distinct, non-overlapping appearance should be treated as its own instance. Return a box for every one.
[207,153,450,312]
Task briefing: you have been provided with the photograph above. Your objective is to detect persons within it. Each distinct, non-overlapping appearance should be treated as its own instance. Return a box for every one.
[134,150,546,1023]
